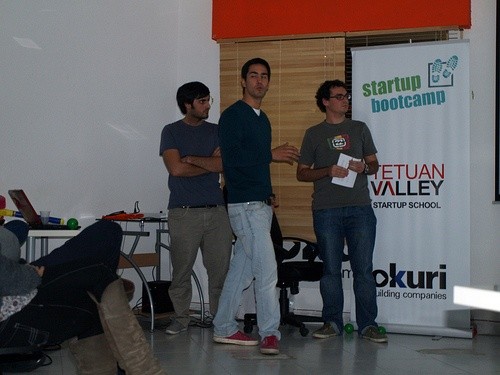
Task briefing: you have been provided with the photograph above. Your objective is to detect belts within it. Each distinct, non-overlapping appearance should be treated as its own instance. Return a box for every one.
[177,204,218,208]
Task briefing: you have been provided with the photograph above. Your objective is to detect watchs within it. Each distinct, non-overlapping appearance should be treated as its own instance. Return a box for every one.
[362,163,369,174]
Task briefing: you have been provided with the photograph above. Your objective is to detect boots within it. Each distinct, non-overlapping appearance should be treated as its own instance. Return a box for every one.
[88,278,162,375]
[68,332,117,375]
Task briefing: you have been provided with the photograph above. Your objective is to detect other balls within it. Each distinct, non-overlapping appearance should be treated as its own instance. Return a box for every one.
[344,324,354,333]
[67,219,78,229]
[378,326,385,336]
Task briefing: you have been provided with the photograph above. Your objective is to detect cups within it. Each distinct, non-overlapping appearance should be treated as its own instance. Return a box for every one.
[40,211,51,225]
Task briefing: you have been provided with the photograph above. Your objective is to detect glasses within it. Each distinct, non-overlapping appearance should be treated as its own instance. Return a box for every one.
[327,94,351,100]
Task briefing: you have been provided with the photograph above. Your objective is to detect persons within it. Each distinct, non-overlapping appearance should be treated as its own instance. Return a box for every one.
[0,223,167,375]
[216,59,301,354]
[297,78,388,343]
[158,81,234,336]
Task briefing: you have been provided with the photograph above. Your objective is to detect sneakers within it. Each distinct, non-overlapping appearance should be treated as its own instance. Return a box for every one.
[215,331,259,346]
[166,322,186,334]
[312,322,336,338]
[260,335,280,354]
[361,326,388,343]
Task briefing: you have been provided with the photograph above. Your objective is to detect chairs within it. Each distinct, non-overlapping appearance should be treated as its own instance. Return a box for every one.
[222,185,323,337]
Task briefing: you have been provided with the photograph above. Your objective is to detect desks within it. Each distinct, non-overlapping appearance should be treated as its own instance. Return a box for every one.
[96,217,204,332]
[26,224,81,264]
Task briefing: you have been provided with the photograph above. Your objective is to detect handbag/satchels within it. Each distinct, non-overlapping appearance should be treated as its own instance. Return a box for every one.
[0,354,46,371]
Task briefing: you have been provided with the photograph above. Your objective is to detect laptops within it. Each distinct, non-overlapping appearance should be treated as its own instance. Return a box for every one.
[8,189,82,231]
